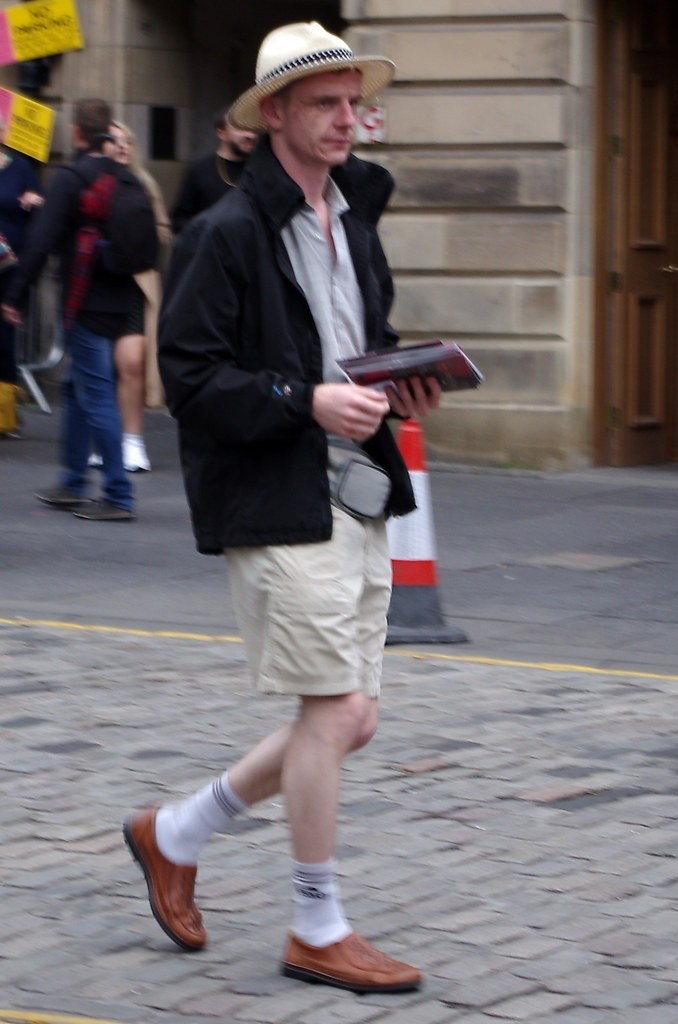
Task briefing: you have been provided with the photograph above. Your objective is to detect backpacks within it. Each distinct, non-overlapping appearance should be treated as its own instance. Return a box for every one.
[62,161,160,280]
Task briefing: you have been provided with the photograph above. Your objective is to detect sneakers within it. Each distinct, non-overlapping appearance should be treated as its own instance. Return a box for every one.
[88,452,103,466]
[121,439,152,472]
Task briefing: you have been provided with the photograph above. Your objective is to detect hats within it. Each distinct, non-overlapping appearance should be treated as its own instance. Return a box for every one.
[226,22,398,131]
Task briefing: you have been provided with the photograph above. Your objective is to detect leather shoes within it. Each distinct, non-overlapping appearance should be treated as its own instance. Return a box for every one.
[277,930,423,995]
[121,802,208,953]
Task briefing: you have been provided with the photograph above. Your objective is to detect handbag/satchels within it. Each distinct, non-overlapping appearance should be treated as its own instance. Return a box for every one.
[325,439,394,523]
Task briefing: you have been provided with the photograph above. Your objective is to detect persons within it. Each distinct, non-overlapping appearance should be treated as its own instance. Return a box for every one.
[0,96,173,526]
[121,18,442,996]
[166,101,260,235]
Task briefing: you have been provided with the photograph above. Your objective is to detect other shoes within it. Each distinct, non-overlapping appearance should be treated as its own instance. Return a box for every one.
[32,488,94,508]
[71,496,137,520]
[7,429,22,440]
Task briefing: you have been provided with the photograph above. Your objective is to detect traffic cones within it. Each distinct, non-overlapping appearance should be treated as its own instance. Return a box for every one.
[385,415,470,647]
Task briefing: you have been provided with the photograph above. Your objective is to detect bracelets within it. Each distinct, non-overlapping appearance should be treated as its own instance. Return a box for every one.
[155,220,172,229]
